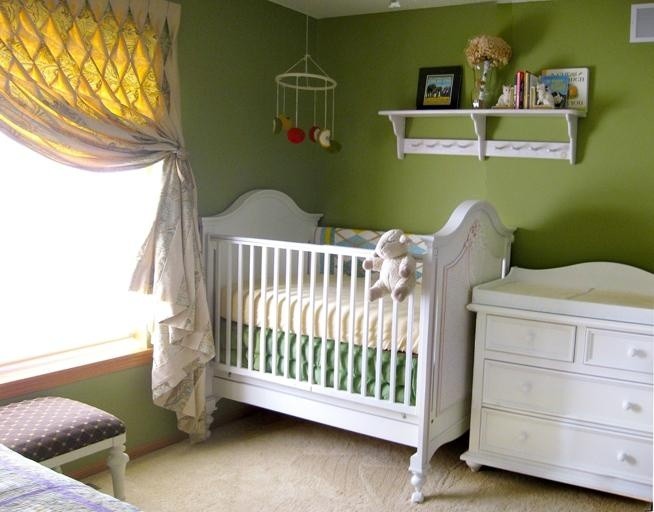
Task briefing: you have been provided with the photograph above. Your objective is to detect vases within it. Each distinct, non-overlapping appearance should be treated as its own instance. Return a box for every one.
[470,59,495,109]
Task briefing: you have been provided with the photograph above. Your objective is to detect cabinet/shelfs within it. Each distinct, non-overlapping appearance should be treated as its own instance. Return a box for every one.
[458,261,653,504]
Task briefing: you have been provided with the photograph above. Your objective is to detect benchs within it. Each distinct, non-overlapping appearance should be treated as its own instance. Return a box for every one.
[1,442,143,512]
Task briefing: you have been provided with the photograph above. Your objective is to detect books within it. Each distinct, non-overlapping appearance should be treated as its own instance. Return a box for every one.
[512,70,559,109]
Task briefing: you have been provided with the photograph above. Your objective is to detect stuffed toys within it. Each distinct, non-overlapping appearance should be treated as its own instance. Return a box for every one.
[358,225,418,302]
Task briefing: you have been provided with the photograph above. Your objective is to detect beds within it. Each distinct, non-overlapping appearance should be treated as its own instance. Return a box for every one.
[196,190,516,503]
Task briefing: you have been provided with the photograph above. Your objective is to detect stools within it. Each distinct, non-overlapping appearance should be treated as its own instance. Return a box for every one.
[0,395,130,501]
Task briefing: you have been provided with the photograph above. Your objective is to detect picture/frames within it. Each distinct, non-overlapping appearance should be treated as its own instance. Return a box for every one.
[416,65,463,109]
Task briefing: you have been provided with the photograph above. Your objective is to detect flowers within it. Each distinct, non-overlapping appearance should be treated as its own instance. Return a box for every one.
[464,35,512,100]
